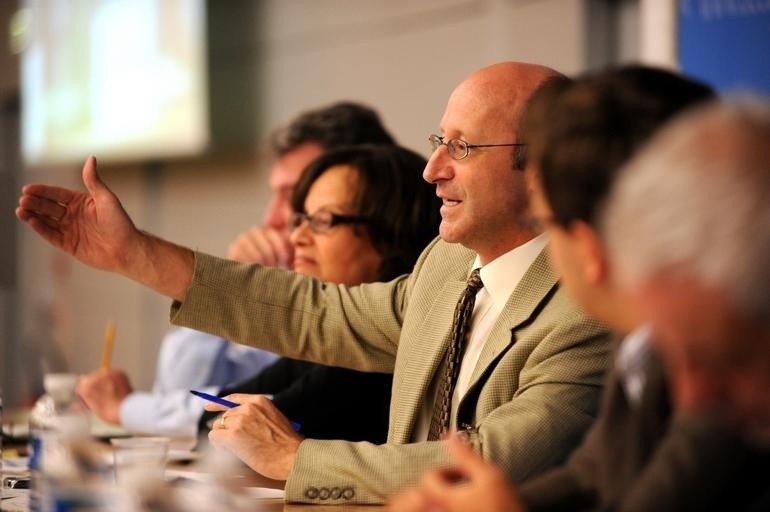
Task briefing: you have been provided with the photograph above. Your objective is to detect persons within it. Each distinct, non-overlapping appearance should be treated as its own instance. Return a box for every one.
[15,60,613,506]
[78,100,395,437]
[380,63,769,511]
[605,90,770,448]
[202,141,439,444]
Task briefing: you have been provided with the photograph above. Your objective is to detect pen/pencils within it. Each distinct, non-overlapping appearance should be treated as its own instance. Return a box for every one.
[190,390,301,433]
[103,320,117,375]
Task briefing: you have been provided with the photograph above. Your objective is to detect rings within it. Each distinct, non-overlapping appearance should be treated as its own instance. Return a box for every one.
[219,417,226,429]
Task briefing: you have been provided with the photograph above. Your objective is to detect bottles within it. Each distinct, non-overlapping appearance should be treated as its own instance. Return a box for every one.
[27,374,93,512]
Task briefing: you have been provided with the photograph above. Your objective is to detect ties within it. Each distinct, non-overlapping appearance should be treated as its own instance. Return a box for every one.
[426,268,484,442]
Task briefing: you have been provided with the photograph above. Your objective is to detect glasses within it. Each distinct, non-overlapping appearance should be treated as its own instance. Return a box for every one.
[286,212,363,234]
[427,133,528,161]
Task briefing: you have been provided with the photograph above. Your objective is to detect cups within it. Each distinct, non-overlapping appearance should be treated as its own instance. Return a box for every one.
[111,437,171,488]
